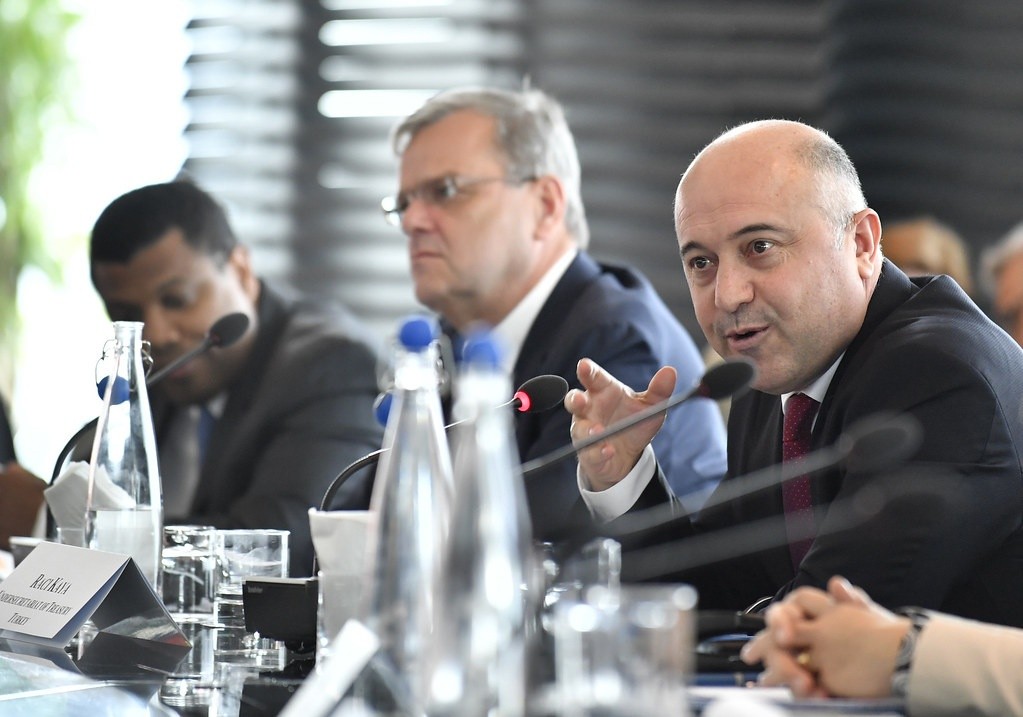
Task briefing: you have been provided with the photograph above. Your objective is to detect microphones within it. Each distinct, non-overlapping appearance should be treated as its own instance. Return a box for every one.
[8,312,249,568]
[243,376,568,653]
[519,362,754,476]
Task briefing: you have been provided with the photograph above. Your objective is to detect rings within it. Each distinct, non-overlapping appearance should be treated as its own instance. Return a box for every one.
[795,651,810,666]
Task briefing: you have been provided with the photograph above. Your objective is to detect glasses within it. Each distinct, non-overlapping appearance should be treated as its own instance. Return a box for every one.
[384,172,537,226]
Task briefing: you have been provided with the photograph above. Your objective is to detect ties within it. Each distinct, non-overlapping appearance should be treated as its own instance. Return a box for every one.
[780,393,816,572]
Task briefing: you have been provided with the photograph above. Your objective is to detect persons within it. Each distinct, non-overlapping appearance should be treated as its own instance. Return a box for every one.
[740,575,1023,717]
[565,120,1023,632]
[0,182,395,653]
[703,218,1023,426]
[380,85,728,598]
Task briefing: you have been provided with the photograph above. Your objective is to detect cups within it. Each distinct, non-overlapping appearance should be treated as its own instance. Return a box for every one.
[161,526,220,627]
[554,583,701,716]
[533,539,621,674]
[209,527,290,629]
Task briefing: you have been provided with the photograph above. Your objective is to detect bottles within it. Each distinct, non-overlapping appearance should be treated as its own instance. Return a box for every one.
[362,320,455,717]
[432,336,540,717]
[82,321,163,626]
